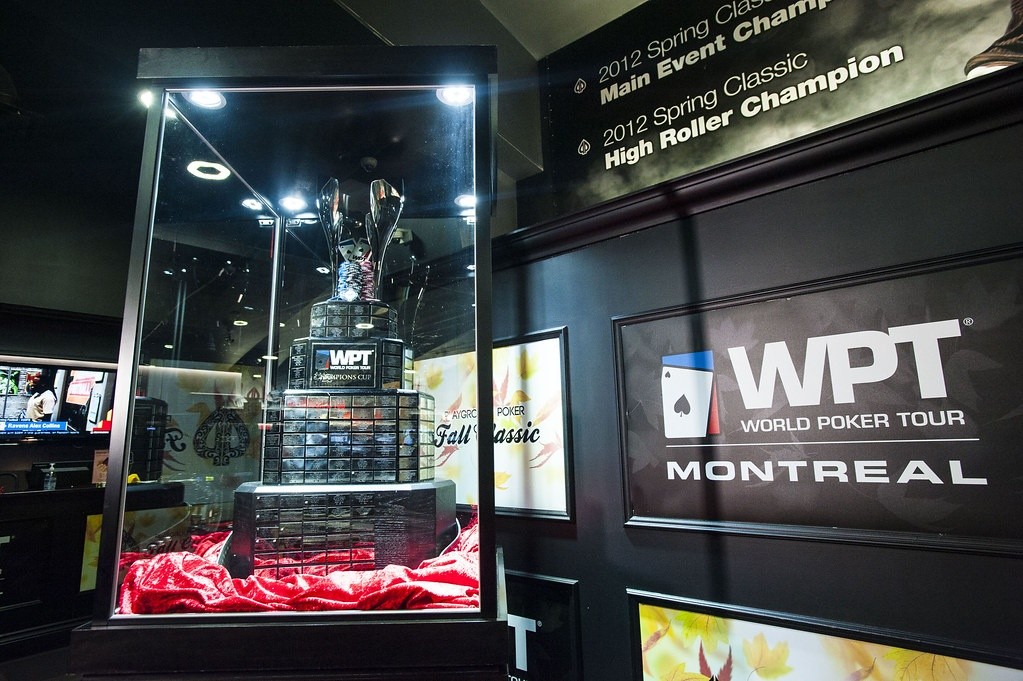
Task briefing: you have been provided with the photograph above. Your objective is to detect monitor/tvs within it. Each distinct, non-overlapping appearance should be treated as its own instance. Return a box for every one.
[27,460,94,491]
[0,361,118,446]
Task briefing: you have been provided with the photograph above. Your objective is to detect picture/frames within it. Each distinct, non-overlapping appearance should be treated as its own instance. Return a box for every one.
[504,568,583,681]
[624,588,1023,681]
[611,241,1023,559]
[413,324,577,525]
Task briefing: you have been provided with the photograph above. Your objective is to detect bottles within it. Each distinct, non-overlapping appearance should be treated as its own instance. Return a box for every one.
[44,464,57,490]
[196,476,217,504]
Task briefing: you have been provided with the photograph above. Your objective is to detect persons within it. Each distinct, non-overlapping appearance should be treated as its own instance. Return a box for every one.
[25,375,56,422]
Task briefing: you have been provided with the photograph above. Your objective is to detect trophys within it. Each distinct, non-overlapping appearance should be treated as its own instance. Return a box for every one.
[232,177,459,580]
